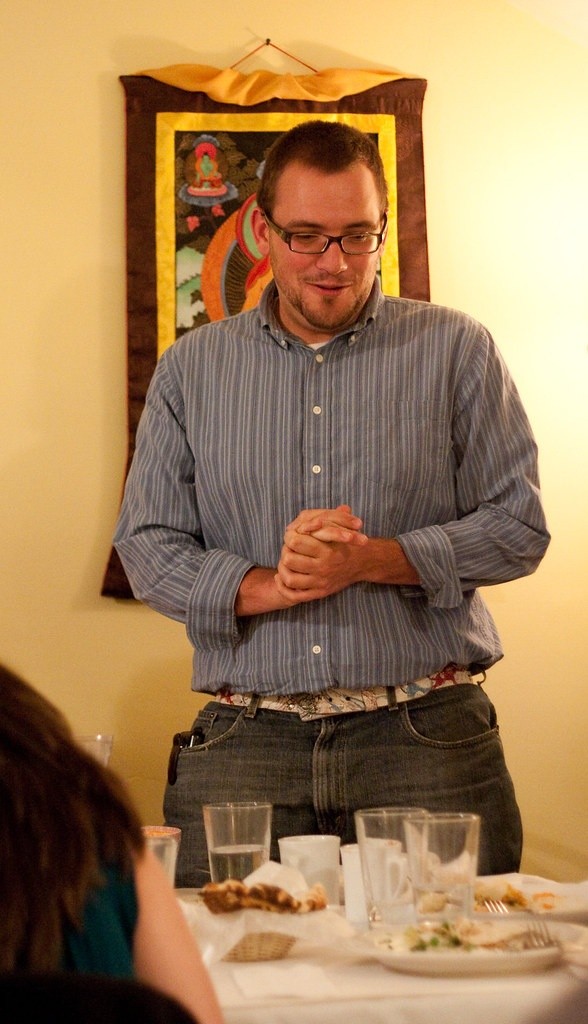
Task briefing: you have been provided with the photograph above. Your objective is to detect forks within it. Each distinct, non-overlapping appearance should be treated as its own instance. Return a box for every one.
[524,920,551,948]
[484,899,509,915]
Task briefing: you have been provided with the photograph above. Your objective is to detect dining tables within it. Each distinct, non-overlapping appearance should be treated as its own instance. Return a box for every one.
[160,877,588,1024]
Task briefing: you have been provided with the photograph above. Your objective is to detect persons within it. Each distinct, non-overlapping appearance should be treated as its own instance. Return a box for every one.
[1,662,224,1024]
[112,122,550,888]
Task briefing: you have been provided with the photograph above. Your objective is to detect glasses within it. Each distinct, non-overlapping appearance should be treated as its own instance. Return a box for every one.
[261,209,387,256]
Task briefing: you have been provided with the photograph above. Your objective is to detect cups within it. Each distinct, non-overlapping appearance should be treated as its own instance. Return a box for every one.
[142,825,182,889]
[403,812,482,916]
[354,807,427,929]
[341,839,403,928]
[279,835,341,904]
[202,801,272,883]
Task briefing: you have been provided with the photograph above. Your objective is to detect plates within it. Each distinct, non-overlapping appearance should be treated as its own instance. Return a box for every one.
[471,881,588,919]
[359,920,588,973]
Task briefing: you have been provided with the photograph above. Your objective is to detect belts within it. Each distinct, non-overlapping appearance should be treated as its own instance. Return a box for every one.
[214,664,477,722]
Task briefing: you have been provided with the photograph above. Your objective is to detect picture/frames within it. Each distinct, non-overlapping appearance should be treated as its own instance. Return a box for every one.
[115,72,435,464]
